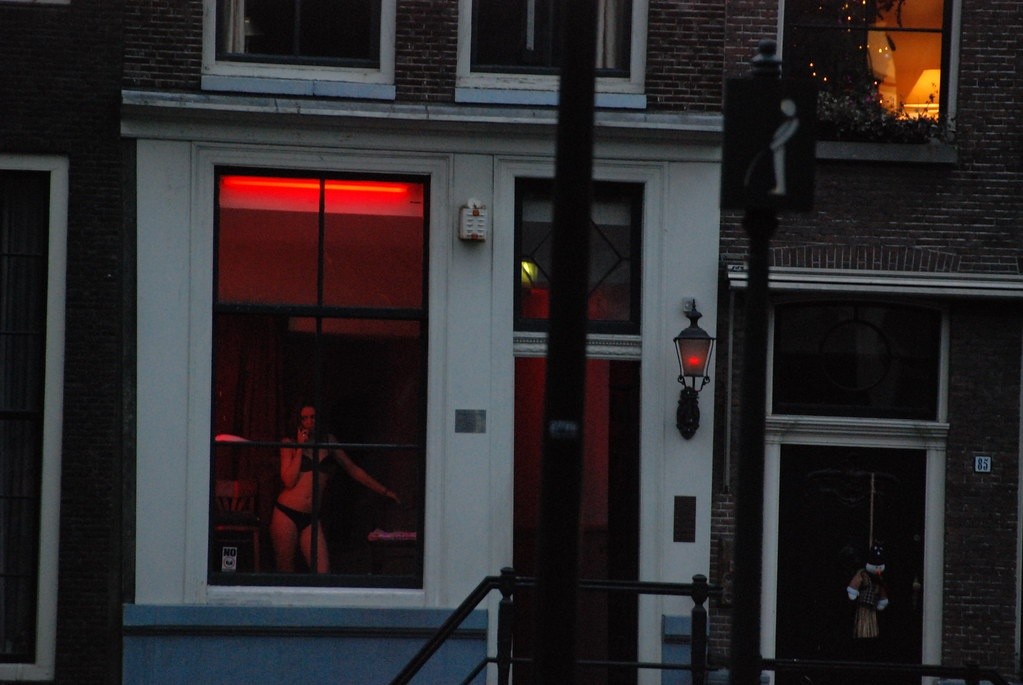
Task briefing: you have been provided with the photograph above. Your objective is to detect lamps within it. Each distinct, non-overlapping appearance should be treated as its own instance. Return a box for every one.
[904,69,942,114]
[522,256,539,290]
[673,298,717,441]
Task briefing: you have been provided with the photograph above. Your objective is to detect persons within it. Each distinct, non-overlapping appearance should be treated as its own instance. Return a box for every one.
[271,403,402,574]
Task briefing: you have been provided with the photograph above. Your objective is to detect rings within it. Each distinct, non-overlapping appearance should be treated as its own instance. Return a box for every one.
[302,434,305,437]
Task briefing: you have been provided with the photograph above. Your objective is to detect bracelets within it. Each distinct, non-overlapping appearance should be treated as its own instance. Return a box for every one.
[382,488,390,496]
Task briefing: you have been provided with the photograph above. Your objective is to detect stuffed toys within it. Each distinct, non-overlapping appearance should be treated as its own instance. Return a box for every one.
[847,544,888,639]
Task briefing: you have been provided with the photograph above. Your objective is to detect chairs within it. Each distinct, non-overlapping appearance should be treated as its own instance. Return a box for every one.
[215,479,261,573]
